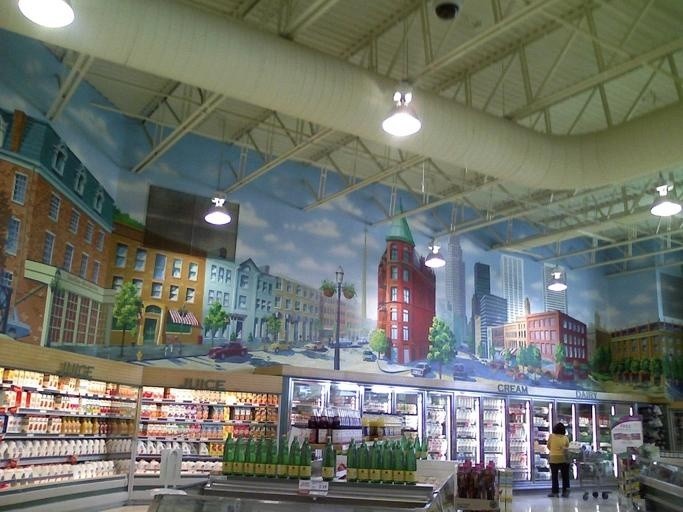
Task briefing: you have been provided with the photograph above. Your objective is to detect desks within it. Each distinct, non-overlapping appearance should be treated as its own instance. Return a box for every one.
[204,459,458,511]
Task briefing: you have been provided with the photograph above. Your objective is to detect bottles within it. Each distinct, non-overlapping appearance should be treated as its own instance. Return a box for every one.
[221,433,429,486]
[457,459,497,500]
[61,415,135,435]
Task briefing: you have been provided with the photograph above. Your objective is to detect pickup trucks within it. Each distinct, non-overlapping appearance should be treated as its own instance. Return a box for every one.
[411,361,431,377]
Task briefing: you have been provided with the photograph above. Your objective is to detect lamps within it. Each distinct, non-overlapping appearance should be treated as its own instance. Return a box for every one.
[424,240,445,268]
[202,192,231,225]
[548,265,567,291]
[649,180,682,217]
[382,83,421,137]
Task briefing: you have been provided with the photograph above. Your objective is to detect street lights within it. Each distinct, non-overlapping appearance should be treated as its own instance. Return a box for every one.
[332,264,343,370]
[45,270,61,347]
[179,303,186,355]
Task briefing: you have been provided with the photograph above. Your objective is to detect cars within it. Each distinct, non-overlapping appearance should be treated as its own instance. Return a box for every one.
[508,369,524,381]
[329,338,352,347]
[305,340,324,352]
[270,340,292,353]
[357,337,366,345]
[362,350,373,362]
[4,307,32,339]
[210,341,245,360]
[451,363,465,381]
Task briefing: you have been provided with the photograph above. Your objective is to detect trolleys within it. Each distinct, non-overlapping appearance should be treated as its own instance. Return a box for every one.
[616,456,644,512]
[571,456,614,502]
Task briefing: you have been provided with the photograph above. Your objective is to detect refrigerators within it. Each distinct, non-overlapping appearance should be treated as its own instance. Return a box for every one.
[282,375,669,490]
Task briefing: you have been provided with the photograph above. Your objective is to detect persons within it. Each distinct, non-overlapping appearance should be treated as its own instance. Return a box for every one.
[547,422,569,498]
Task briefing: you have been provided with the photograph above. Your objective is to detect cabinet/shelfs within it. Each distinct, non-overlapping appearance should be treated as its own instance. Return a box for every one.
[0,380,280,494]
